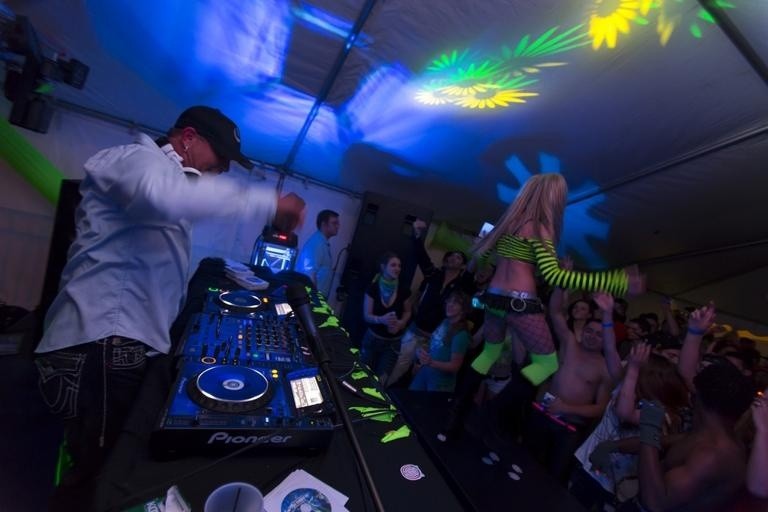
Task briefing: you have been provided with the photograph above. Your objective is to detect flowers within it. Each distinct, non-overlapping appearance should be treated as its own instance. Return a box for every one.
[413,0,735,109]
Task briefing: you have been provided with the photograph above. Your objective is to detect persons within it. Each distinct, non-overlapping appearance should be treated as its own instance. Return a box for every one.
[34,106,306,512]
[293,210,341,303]
[359,174,768,511]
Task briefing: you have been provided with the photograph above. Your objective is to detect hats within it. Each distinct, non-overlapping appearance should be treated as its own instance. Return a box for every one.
[174,106,254,170]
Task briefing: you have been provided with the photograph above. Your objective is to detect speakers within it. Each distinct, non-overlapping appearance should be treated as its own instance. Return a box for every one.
[339,190,436,302]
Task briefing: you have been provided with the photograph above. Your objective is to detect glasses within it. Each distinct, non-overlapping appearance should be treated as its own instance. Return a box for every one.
[445,299,457,304]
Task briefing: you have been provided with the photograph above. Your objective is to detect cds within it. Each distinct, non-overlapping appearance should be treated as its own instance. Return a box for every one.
[280,487,333,511]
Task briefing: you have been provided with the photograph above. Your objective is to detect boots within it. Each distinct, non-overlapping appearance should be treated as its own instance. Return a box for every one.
[440,366,487,439]
[494,370,539,445]
